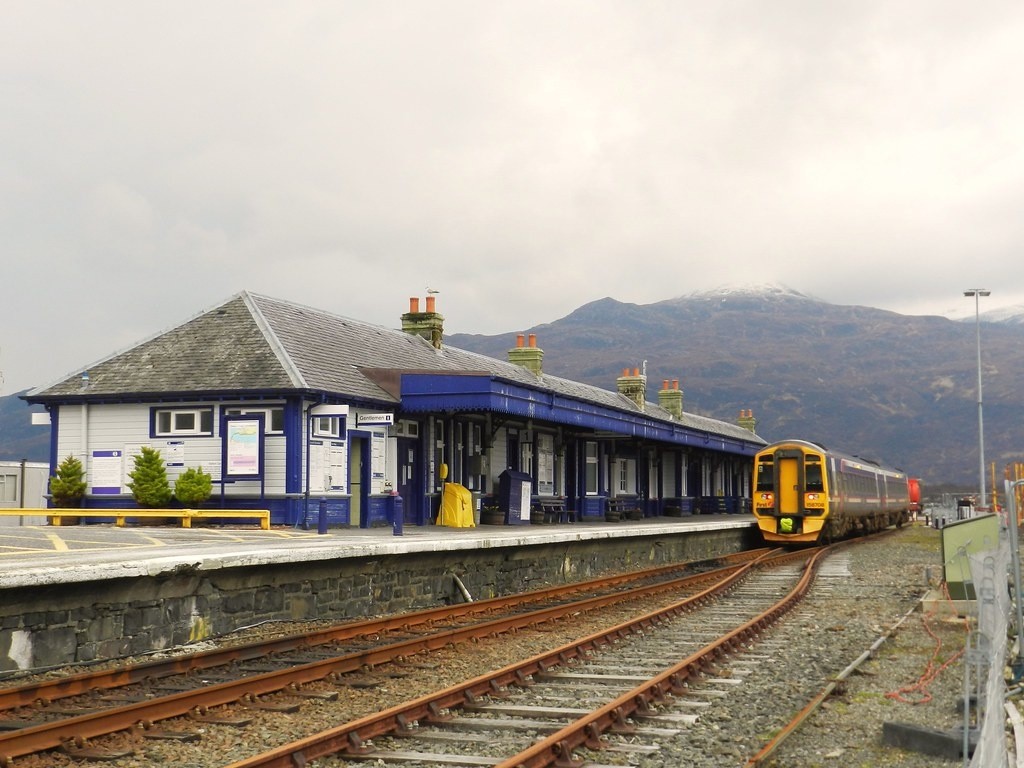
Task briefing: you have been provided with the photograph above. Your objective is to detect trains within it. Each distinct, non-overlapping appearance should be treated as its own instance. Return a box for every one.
[752,439,911,547]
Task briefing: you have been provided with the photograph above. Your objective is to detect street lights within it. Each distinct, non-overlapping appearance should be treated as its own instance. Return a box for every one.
[963,287,991,508]
